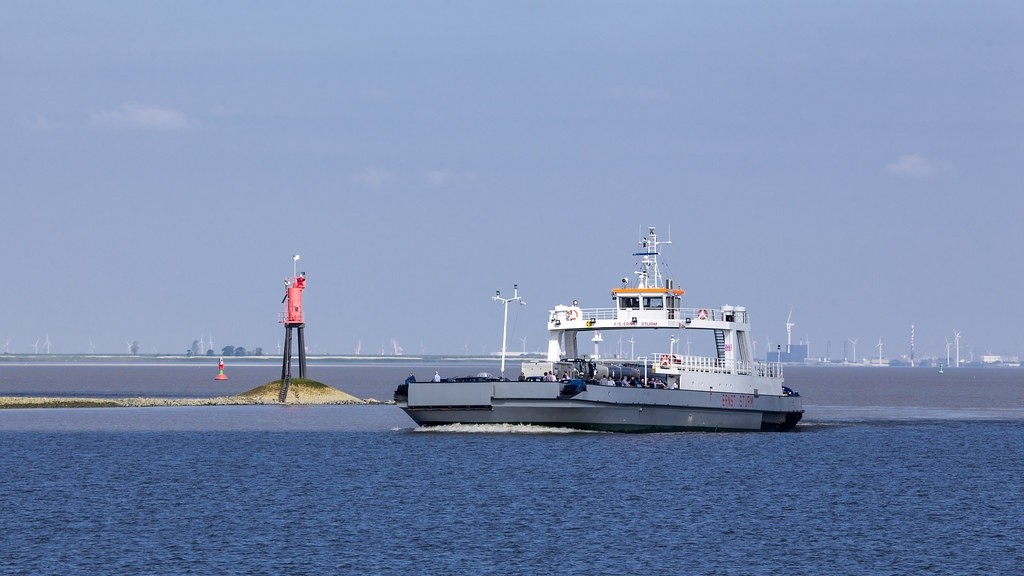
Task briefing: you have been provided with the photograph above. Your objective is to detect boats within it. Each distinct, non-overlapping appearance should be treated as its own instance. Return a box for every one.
[395,223,805,433]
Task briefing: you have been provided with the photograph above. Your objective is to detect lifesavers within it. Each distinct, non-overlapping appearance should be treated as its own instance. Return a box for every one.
[697,309,708,320]
[568,310,578,320]
[659,354,673,366]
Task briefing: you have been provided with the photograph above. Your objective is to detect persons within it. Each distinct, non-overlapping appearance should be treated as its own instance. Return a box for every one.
[587,374,679,389]
[405,372,416,384]
[433,371,440,383]
[544,370,571,383]
[518,372,526,381]
